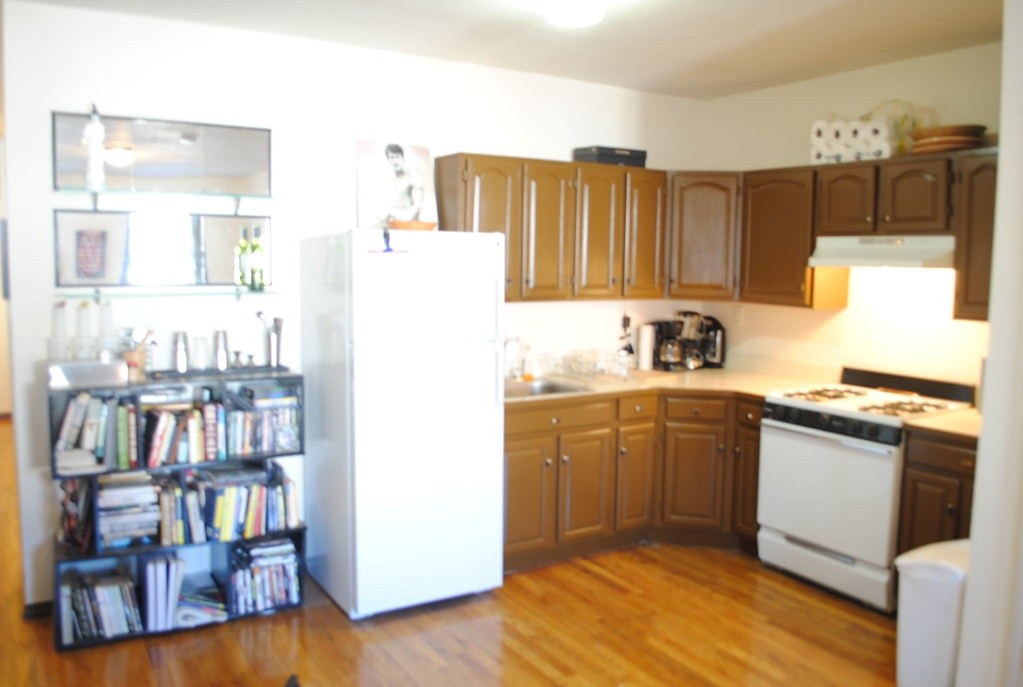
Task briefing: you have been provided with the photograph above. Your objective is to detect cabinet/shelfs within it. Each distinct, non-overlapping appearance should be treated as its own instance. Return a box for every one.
[897,429,976,556]
[503,391,766,577]
[433,143,999,320]
[45,366,306,650]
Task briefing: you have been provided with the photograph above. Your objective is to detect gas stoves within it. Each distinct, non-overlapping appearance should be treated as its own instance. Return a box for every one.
[760,366,976,451]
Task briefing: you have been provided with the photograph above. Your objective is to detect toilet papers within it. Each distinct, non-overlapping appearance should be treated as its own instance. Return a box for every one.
[637,324,655,372]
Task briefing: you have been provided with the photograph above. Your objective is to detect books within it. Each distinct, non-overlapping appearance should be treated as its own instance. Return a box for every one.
[53,390,140,477]
[60,550,231,645]
[96,458,301,548]
[138,385,302,468]
[230,534,301,613]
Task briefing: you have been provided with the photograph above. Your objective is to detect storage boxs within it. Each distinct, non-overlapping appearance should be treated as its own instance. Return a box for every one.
[44,358,129,389]
[573,147,647,169]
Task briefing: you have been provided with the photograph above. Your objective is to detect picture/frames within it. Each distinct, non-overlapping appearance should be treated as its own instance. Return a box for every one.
[50,114,272,199]
[196,215,271,285]
[52,208,131,287]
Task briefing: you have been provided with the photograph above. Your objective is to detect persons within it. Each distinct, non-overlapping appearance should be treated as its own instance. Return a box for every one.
[385,142,431,224]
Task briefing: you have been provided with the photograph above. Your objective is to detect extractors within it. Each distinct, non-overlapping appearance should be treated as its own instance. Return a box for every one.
[807,235,956,269]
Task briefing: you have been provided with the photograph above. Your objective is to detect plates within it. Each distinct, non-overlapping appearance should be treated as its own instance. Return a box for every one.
[909,125,987,154]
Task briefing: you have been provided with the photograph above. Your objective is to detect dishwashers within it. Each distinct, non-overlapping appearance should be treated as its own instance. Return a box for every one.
[756,418,906,613]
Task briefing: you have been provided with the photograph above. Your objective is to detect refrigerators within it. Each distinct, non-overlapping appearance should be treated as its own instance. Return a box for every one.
[300,229,505,621]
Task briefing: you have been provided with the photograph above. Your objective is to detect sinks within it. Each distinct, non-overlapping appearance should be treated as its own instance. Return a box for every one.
[505,375,593,399]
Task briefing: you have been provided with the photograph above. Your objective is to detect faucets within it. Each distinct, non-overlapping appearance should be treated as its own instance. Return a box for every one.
[502,335,525,379]
